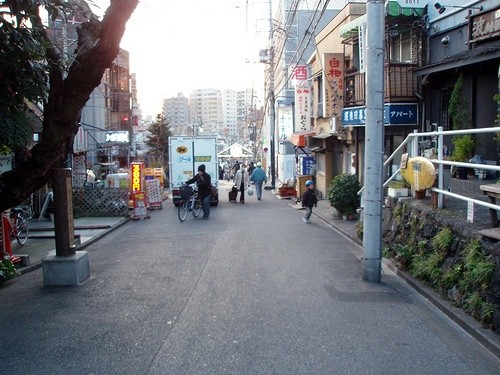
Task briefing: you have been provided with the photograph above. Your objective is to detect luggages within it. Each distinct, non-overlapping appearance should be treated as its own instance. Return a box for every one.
[229,185,237,202]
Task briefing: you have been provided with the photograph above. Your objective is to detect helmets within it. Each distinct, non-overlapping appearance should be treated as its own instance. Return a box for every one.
[305,180,314,185]
[257,162,262,167]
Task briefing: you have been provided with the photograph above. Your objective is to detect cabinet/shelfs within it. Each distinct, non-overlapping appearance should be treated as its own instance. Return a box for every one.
[297,175,313,201]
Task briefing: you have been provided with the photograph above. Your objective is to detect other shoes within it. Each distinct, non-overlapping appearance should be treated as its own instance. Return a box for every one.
[258,196,261,200]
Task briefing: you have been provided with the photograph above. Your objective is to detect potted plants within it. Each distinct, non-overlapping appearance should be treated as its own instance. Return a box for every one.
[328,173,363,221]
[448,70,500,180]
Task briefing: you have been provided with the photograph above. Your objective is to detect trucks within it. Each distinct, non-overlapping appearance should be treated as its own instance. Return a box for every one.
[168,135,219,208]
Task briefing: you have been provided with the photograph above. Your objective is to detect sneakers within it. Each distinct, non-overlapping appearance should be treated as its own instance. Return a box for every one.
[201,214,205,219]
[204,215,208,220]
[302,218,311,224]
[240,200,244,203]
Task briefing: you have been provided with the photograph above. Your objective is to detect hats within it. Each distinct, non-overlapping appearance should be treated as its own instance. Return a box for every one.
[198,164,205,171]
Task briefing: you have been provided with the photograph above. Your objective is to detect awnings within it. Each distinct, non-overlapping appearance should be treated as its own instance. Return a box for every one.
[339,0,426,39]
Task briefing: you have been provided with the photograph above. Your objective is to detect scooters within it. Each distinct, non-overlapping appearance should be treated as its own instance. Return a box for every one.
[83,163,119,189]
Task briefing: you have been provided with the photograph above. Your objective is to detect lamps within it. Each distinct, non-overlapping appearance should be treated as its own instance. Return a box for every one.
[433,2,484,14]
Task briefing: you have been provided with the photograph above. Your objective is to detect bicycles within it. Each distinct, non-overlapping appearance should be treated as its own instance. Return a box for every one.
[177,183,204,222]
[0,205,29,246]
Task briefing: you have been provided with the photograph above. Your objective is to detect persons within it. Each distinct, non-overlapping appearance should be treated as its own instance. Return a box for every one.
[234,163,249,204]
[249,161,267,200]
[232,161,240,182]
[218,166,224,180]
[247,162,256,175]
[184,164,212,220]
[302,180,318,224]
